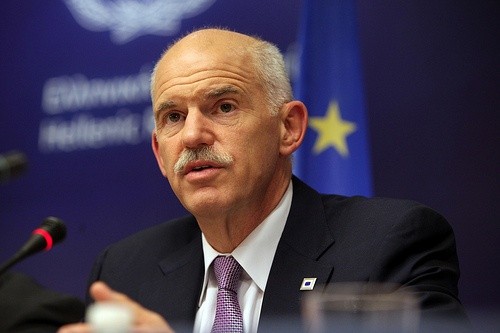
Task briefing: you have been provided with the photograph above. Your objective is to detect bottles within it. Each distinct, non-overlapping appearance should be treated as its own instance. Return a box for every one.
[86,302,136,333]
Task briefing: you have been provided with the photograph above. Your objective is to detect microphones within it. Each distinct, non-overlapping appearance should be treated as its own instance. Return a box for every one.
[0,216,67,276]
[0,152,25,182]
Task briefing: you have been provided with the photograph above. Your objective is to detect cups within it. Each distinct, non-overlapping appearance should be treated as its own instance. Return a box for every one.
[302,281,420,332]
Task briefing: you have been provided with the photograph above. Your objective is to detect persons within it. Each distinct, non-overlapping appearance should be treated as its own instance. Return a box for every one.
[57,28,471,333]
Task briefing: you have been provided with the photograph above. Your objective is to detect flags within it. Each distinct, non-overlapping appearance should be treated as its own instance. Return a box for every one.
[292,0,373,202]
